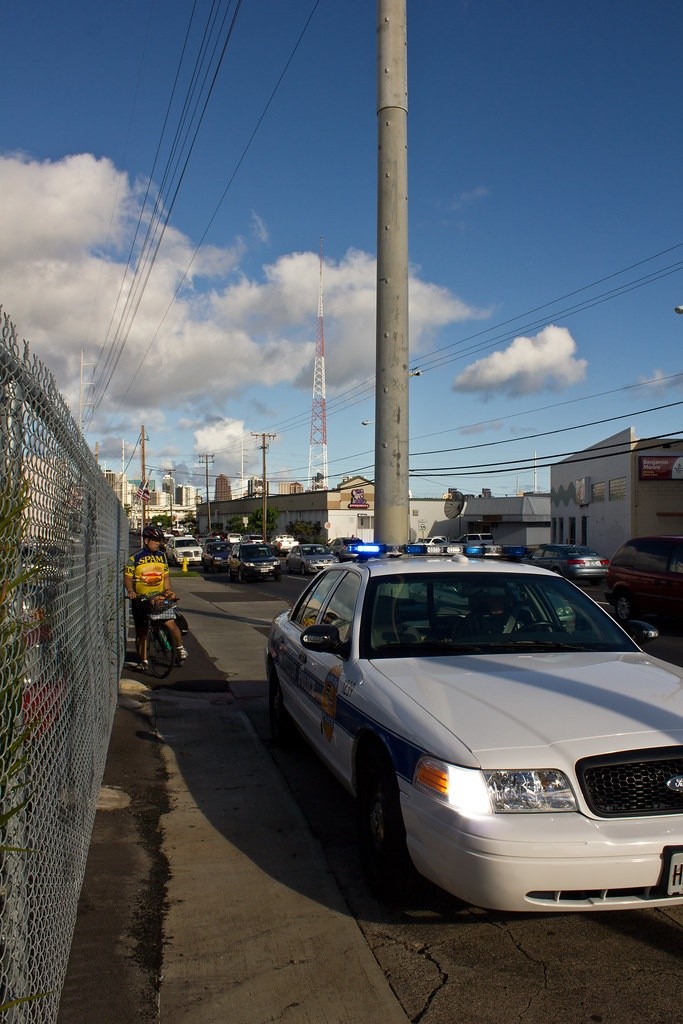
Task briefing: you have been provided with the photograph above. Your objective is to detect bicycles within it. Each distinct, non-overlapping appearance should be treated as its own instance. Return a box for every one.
[123,592,181,680]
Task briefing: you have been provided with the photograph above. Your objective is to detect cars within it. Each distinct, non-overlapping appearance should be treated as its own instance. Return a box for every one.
[408,580,576,637]
[165,536,203,567]
[263,542,683,917]
[0,542,88,748]
[160,528,266,546]
[285,544,339,575]
[129,528,141,535]
[413,538,448,546]
[519,544,609,586]
[325,537,368,562]
[201,542,232,574]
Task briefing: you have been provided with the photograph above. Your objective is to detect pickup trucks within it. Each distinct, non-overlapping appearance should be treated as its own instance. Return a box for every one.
[270,535,299,557]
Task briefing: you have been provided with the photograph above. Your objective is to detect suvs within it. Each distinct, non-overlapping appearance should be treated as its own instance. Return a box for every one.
[227,543,281,584]
[603,535,683,624]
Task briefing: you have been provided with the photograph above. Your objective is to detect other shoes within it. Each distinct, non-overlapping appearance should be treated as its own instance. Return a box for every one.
[178,645,188,660]
[130,660,148,670]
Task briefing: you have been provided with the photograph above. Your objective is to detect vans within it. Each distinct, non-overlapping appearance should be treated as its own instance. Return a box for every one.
[451,533,495,546]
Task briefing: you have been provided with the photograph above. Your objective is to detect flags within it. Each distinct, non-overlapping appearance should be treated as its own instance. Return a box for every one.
[143,479,150,499]
[137,481,149,502]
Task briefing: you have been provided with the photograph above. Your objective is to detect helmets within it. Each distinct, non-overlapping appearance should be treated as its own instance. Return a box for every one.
[141,526,165,539]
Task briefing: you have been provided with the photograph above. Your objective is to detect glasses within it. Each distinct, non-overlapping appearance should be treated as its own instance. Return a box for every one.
[150,537,162,542]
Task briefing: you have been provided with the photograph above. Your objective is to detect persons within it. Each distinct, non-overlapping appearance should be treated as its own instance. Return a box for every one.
[186,541,325,558]
[451,582,542,644]
[123,527,188,671]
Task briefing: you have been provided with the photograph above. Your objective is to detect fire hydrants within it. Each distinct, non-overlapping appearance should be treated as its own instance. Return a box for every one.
[180,557,189,573]
